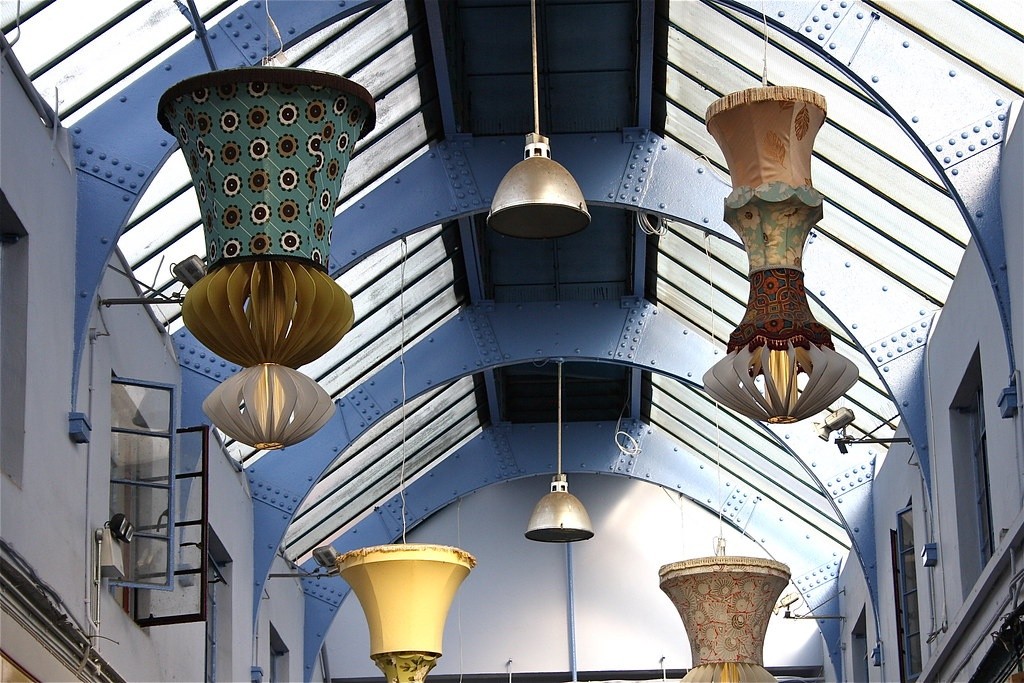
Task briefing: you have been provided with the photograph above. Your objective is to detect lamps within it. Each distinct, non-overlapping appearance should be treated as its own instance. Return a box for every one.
[525,363,596,545]
[104,514,136,543]
[95,253,209,308]
[266,546,342,580]
[484,0,591,242]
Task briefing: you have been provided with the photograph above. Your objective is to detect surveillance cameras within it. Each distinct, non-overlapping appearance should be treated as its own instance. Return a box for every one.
[773,592,798,615]
[812,409,853,441]
